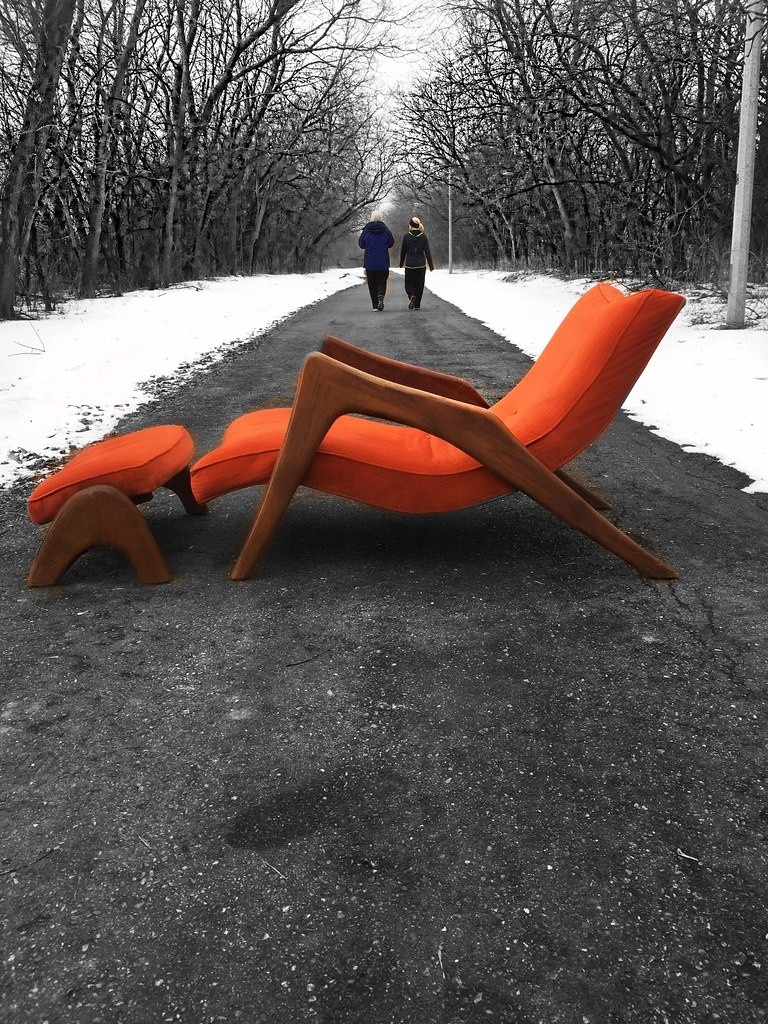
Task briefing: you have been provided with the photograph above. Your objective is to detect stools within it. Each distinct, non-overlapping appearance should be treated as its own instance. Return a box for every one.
[25,422,207,590]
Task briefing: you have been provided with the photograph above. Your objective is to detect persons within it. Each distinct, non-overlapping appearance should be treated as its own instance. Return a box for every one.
[400,217,434,310]
[357,210,395,312]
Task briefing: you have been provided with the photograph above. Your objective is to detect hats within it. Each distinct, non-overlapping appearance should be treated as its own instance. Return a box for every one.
[371,210,382,221]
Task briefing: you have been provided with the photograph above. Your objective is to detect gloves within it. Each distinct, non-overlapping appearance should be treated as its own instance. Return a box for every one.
[430,266,434,271]
[400,264,403,268]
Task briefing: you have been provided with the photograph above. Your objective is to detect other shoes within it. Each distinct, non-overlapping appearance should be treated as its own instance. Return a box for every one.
[377,292,385,311]
[415,307,420,310]
[373,306,378,312]
[408,294,416,309]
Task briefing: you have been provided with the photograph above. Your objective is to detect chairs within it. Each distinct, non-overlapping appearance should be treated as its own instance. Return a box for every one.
[186,282,684,584]
